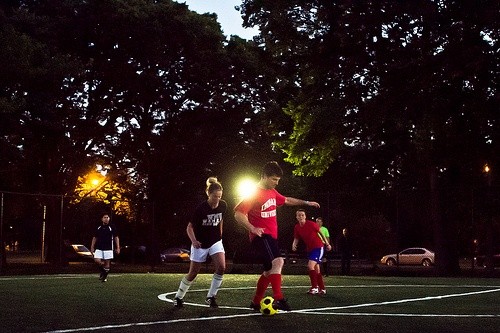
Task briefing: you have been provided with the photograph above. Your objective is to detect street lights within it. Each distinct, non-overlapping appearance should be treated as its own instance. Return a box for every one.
[58,176,100,267]
[235,177,261,270]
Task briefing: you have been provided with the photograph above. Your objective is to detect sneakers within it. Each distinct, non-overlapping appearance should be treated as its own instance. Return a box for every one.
[174,297,185,309]
[318,289,327,296]
[306,288,319,294]
[205,296,219,308]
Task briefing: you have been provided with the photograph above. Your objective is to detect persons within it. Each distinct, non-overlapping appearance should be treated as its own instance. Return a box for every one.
[317,217,331,276]
[337,228,354,275]
[292,210,332,296]
[91,212,120,282]
[173,177,228,309]
[234,162,320,311]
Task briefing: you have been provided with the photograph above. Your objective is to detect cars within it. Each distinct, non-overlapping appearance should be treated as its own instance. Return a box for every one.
[71,244,93,260]
[380,248,435,267]
[474,254,500,270]
[159,248,190,262]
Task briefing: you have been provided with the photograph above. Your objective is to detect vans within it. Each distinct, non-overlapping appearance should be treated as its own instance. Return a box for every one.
[281,253,299,264]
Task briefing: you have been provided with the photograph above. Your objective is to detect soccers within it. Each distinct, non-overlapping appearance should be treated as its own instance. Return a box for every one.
[260,296,279,314]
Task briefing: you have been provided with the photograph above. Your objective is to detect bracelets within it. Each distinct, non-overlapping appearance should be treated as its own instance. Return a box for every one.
[326,244,330,246]
[303,201,308,206]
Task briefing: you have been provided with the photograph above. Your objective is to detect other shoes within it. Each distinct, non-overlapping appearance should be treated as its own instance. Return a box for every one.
[102,278,107,282]
[324,274,328,277]
[249,302,261,313]
[99,275,103,280]
[273,298,292,311]
